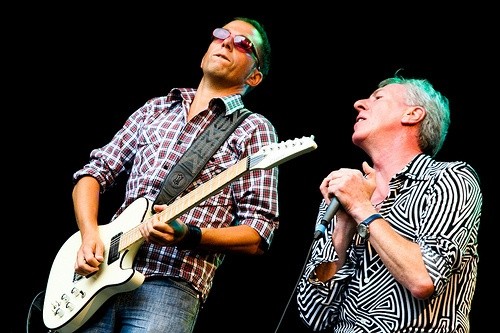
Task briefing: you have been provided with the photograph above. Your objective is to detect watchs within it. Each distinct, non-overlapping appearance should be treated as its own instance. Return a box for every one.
[356,214,384,239]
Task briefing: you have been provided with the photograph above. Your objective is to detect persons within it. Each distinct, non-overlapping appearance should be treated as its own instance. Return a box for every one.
[72,17,279,333]
[297,75,483,333]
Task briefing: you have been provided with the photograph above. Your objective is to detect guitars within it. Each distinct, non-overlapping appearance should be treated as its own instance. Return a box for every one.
[43,134,319,333]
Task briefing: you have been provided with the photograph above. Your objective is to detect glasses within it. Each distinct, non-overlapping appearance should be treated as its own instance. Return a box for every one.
[210,28,261,71]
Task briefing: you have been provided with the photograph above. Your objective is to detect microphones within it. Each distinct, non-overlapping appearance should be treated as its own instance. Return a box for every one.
[315,198,340,240]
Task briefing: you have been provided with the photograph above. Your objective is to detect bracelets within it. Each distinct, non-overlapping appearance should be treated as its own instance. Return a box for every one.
[177,224,202,251]
